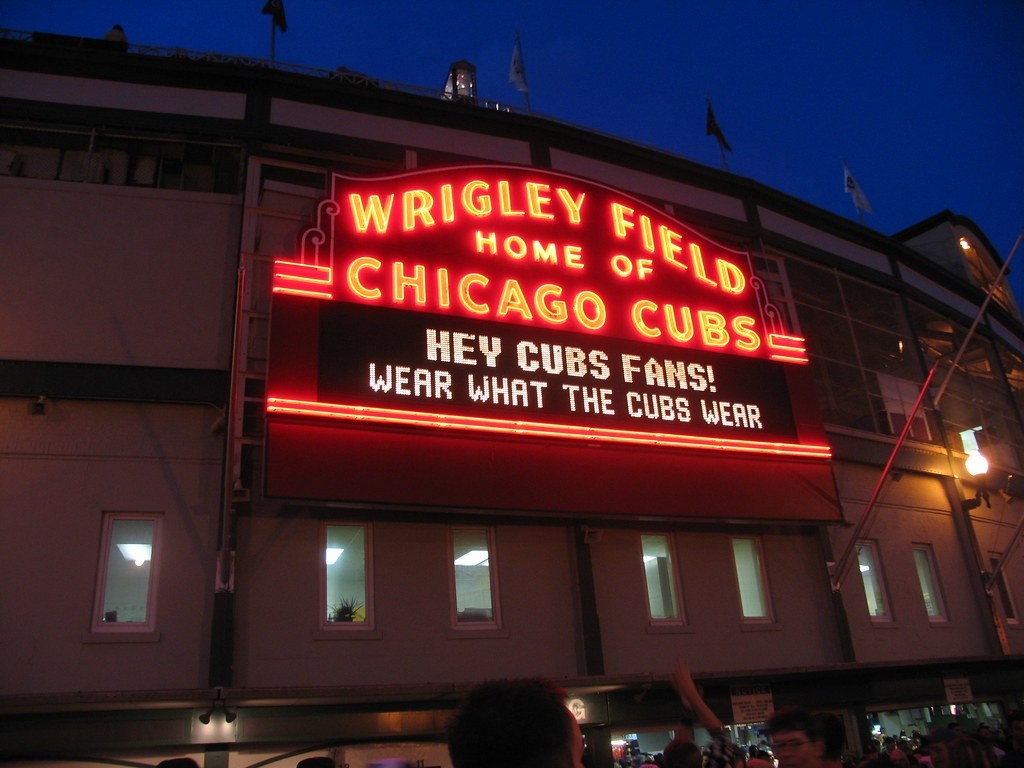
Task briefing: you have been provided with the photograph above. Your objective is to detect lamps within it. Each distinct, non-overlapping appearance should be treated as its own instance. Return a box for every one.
[962,448,993,510]
[198,698,236,725]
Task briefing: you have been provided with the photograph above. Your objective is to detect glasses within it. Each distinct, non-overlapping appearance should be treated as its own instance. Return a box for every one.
[771,741,811,754]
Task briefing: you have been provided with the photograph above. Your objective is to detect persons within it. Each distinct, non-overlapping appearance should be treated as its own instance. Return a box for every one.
[445,678,586,768]
[615,661,1024,768]
[104,25,126,43]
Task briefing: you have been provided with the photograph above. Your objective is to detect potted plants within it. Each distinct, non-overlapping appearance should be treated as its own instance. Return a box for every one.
[330,593,365,622]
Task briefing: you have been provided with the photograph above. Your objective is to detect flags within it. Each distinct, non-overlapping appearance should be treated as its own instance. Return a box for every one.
[706,103,731,151]
[261,0,286,33]
[844,168,872,214]
[508,39,529,94]
